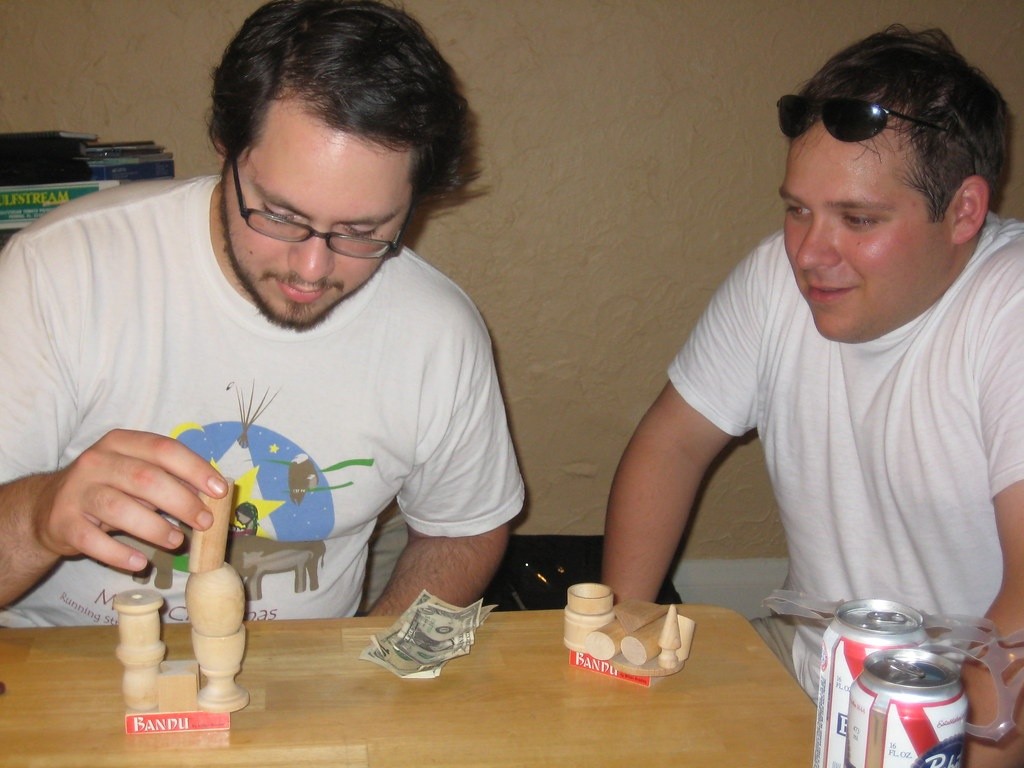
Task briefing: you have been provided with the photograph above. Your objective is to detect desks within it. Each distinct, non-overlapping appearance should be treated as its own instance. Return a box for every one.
[0,604,817,768]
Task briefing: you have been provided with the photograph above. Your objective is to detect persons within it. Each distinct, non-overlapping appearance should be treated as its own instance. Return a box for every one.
[1,0,526,629]
[601,22,1024,768]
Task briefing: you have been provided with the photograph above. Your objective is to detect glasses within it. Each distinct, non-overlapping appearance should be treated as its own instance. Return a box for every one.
[776,94,961,142]
[231,151,419,260]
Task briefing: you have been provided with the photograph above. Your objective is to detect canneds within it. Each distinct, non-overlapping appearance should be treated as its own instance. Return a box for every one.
[813,599,927,768]
[843,648,970,768]
